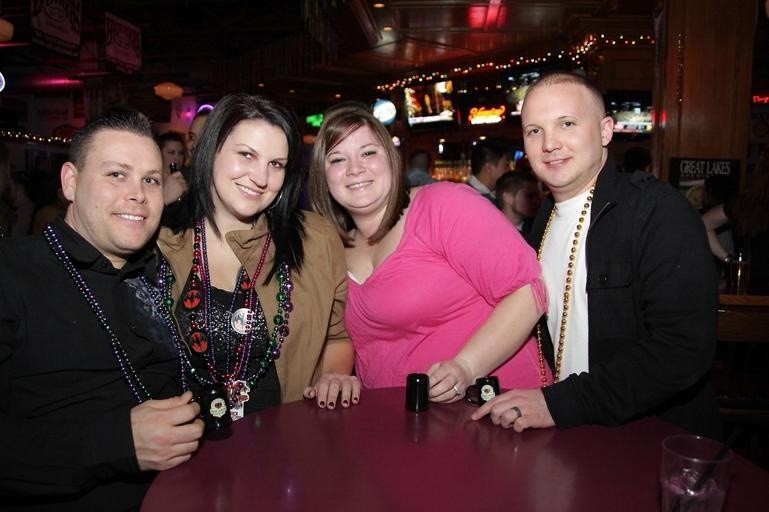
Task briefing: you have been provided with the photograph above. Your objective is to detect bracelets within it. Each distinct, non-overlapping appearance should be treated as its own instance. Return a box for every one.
[724,254,733,263]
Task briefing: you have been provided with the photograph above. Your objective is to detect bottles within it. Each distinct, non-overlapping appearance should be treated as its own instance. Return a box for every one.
[430,159,471,180]
[170,162,188,204]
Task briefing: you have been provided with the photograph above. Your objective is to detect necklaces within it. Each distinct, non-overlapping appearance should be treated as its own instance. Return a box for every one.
[164,216,294,409]
[536,181,596,388]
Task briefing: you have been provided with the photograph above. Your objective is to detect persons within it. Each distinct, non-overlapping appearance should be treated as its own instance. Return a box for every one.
[159,113,208,226]
[405,138,543,237]
[702,176,768,295]
[155,91,363,423]
[469,70,721,434]
[306,102,553,405]
[1,112,205,511]
[620,148,653,173]
[3,144,68,239]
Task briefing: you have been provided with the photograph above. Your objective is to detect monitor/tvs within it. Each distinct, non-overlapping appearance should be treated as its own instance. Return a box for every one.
[502,69,541,116]
[404,80,455,126]
[607,97,652,139]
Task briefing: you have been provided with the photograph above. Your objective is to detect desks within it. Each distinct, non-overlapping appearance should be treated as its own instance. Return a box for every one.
[139,386,768,512]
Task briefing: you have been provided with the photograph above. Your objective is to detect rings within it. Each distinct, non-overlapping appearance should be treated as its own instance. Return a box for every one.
[512,406,522,419]
[453,387,459,395]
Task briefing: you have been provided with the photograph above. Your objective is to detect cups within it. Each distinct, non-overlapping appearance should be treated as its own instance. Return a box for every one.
[187,382,234,440]
[661,434,736,512]
[465,375,499,408]
[404,410,429,445]
[729,248,753,296]
[405,371,431,412]
